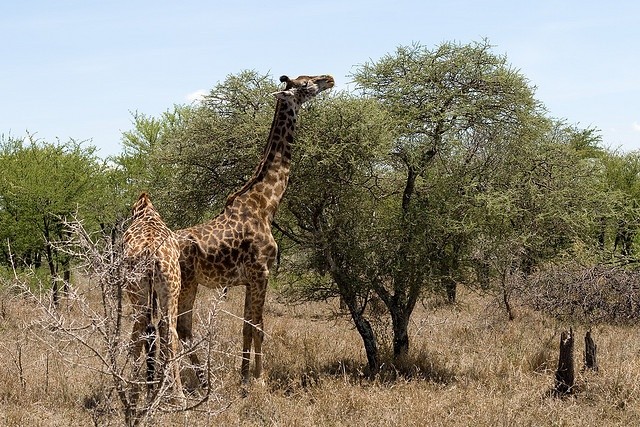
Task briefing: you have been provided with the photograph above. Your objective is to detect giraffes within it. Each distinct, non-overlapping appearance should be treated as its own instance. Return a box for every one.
[120,192,186,406]
[172,75,334,379]
